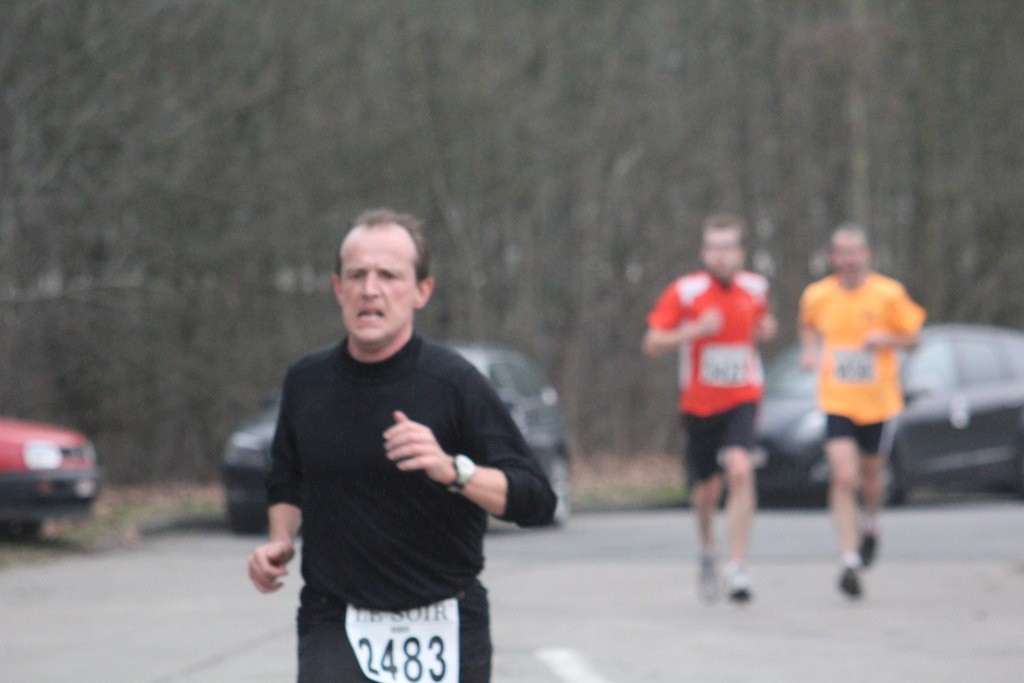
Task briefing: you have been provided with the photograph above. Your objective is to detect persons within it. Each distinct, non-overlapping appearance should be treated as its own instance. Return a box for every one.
[642,216,776,602]
[247,208,559,683]
[798,227,925,596]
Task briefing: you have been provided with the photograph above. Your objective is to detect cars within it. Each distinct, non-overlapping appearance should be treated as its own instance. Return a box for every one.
[221,344,570,539]
[0,416,104,546]
[713,323,1024,510]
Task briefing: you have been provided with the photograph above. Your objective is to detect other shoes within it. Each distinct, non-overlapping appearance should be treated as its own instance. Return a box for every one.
[728,562,749,598]
[698,560,718,598]
[839,567,861,597]
[862,536,876,562]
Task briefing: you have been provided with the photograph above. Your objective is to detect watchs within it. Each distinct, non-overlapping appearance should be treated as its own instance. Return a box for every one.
[446,454,474,493]
